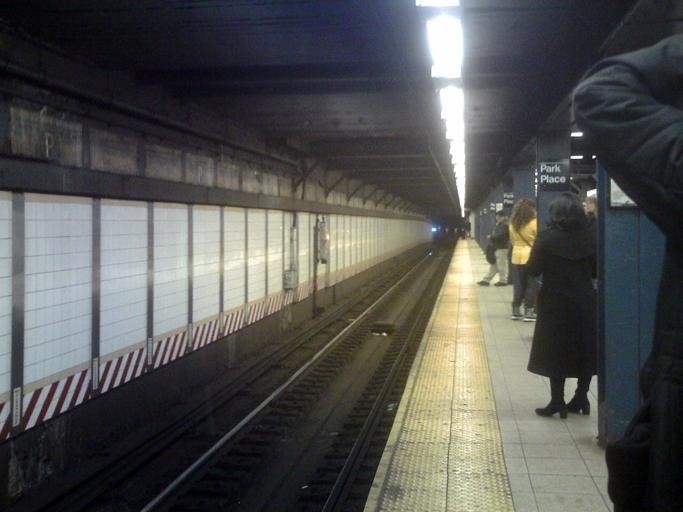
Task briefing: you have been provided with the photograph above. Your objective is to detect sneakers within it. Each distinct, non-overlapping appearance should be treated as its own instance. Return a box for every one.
[476,280,507,287]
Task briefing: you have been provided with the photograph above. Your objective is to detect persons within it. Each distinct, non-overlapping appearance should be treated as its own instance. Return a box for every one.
[476,209,511,287]
[510,196,540,320]
[578,194,598,223]
[524,185,601,415]
[569,23,680,510]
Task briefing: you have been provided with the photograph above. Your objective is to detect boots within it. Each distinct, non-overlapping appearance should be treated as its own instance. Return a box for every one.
[522,305,536,322]
[533,374,567,419]
[567,372,591,416]
[510,304,523,319]
